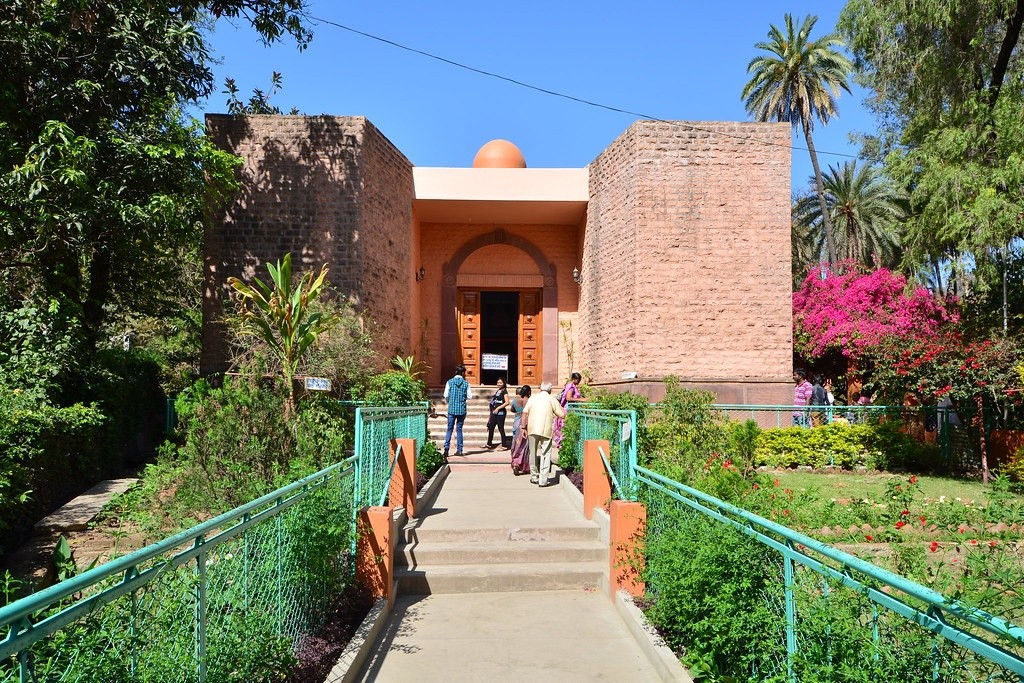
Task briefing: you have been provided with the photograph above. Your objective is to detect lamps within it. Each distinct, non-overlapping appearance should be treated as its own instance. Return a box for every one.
[572,265,583,287]
[415,265,425,284]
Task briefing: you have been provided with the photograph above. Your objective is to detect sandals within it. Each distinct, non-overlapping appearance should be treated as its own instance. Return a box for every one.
[481,444,491,448]
[501,446,507,451]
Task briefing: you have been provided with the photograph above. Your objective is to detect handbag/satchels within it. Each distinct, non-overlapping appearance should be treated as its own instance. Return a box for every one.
[557,389,565,400]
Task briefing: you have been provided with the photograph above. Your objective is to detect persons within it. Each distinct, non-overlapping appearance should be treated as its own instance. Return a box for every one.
[554,372,587,450]
[792,368,814,427]
[809,375,827,427]
[480,377,509,452]
[509,385,531,477]
[440,365,473,457]
[520,382,565,488]
[852,392,870,405]
[824,386,834,425]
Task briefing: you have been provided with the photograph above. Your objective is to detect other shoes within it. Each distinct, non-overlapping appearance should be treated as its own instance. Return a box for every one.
[539,485,548,487]
[454,452,462,455]
[443,452,448,456]
[514,467,519,476]
[530,478,538,484]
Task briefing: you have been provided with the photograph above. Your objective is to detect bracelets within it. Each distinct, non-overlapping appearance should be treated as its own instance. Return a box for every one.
[521,427,526,430]
